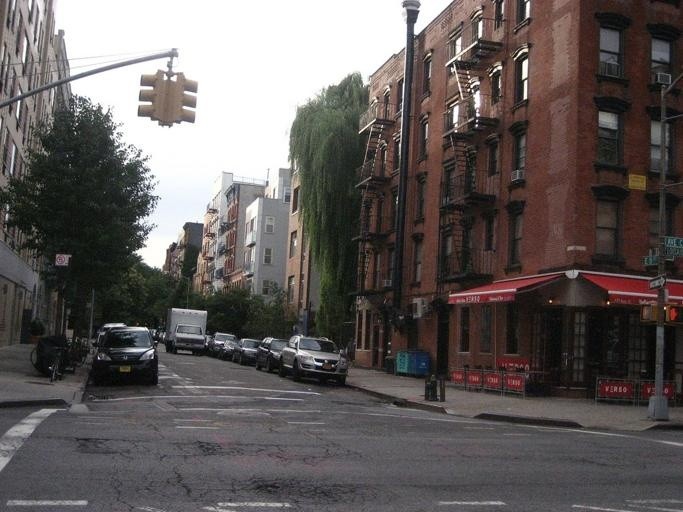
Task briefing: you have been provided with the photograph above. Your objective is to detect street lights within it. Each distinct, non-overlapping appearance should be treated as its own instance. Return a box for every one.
[391,1,419,304]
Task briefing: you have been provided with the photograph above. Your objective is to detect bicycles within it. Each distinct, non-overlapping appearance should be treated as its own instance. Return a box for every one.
[30,334,89,383]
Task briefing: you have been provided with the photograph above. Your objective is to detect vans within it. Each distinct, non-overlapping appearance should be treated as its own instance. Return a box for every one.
[278,334,348,386]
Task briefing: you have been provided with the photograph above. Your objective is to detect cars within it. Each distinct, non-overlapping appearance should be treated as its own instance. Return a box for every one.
[150,328,167,344]
[91,322,159,387]
[256,336,290,372]
[205,331,261,366]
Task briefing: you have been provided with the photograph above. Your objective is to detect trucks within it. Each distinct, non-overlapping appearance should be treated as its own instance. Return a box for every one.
[164,307,208,356]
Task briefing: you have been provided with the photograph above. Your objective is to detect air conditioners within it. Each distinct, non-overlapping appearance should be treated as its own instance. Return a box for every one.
[382,280,392,287]
[511,169,525,182]
[656,73,671,85]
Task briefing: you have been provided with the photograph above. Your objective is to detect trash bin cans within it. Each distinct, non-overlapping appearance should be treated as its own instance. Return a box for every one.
[385,351,430,378]
[424,377,437,401]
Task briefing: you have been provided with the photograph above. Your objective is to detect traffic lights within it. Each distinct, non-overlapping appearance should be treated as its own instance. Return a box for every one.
[138,70,198,128]
[664,304,683,322]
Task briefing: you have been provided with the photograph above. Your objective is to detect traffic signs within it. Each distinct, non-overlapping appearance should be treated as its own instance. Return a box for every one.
[639,236,682,267]
